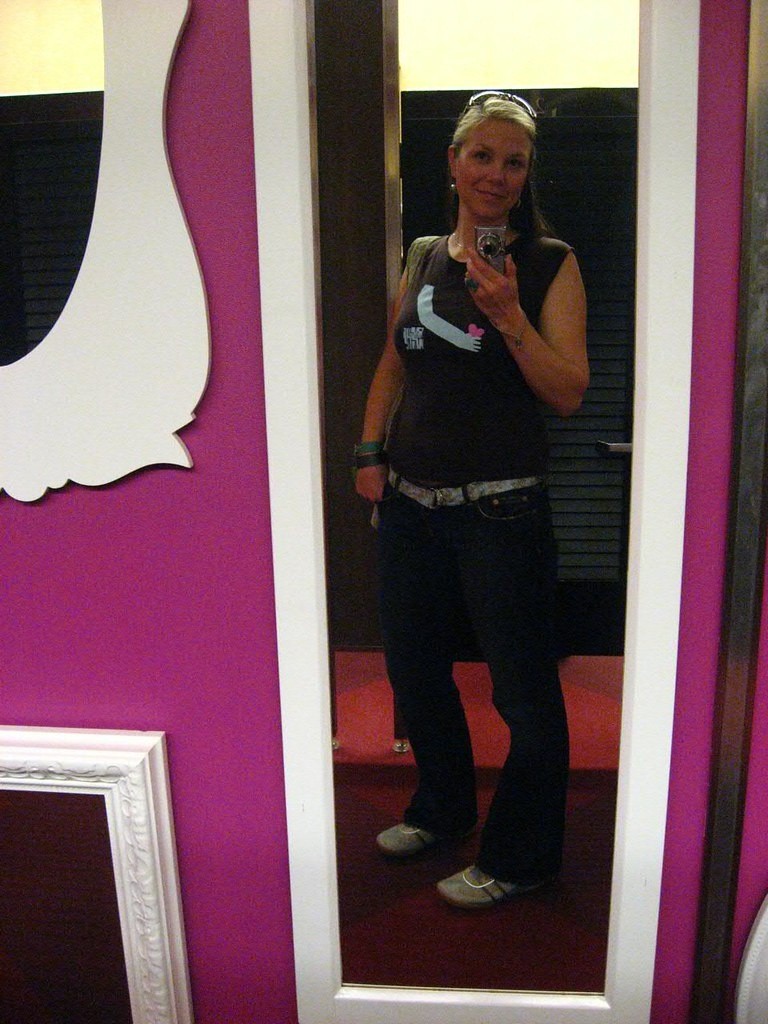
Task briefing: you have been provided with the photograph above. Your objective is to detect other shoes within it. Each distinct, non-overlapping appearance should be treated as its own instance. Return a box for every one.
[436,863,544,909]
[376,822,436,856]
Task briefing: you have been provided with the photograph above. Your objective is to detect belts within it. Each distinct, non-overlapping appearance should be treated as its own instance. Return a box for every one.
[388,470,544,510]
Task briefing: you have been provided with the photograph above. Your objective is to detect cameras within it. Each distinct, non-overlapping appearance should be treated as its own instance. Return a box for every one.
[474,226,505,276]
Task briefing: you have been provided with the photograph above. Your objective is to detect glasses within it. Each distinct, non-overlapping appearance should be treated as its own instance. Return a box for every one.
[463,91,537,120]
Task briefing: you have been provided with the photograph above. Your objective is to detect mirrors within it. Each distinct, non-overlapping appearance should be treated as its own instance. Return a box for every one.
[0,724,197,1024]
[1,0,214,505]
[247,0,702,1024]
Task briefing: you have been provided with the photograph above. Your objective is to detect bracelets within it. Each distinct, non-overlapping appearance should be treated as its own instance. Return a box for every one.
[356,453,384,469]
[354,442,382,454]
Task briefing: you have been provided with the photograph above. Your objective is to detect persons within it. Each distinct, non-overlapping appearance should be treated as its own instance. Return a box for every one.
[355,91,589,908]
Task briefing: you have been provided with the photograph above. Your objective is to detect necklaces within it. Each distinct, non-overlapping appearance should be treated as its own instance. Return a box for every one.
[450,232,465,250]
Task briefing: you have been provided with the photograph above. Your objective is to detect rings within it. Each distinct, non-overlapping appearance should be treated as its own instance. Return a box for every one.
[465,278,480,291]
